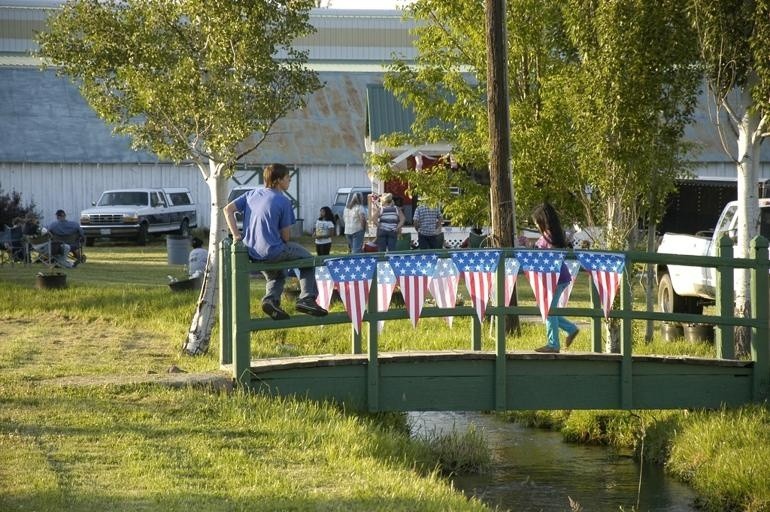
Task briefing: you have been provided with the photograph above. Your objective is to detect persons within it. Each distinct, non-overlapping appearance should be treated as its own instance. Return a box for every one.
[412,204,444,249]
[311,207,336,256]
[12,212,46,263]
[530,205,579,353]
[372,193,406,252]
[342,192,366,254]
[47,210,86,268]
[222,163,328,321]
[189,237,210,278]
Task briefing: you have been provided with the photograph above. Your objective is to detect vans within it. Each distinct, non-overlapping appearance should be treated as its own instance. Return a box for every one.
[78,187,198,246]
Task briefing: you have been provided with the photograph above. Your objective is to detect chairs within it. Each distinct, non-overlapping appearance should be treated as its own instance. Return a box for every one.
[0,224,86,266]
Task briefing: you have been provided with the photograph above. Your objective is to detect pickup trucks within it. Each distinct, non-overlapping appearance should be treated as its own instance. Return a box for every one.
[651,198,770,323]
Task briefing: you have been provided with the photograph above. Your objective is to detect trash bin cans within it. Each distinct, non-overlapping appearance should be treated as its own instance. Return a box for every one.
[167,234,191,264]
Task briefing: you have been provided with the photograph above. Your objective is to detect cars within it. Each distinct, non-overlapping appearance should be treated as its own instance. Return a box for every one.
[332,185,372,236]
[225,184,265,238]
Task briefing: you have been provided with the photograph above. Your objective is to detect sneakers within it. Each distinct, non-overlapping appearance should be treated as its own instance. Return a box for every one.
[534,329,580,353]
[295,296,329,316]
[261,298,291,321]
